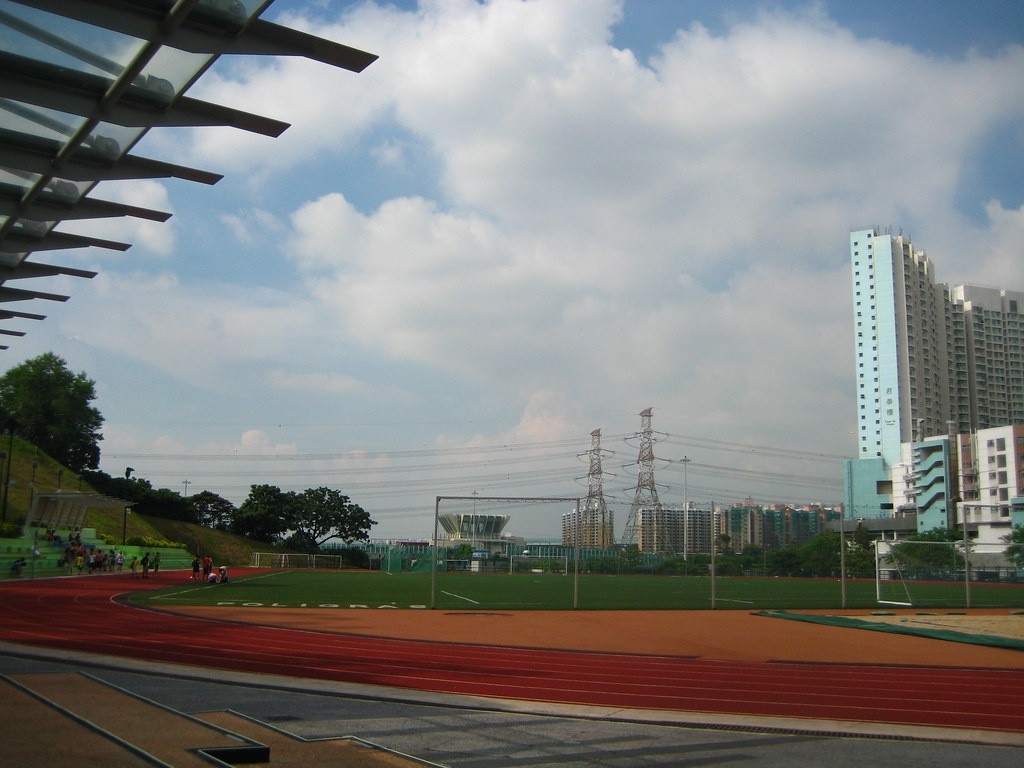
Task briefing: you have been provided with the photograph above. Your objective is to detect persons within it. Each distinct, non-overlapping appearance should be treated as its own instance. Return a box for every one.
[11,526,161,578]
[190,553,229,585]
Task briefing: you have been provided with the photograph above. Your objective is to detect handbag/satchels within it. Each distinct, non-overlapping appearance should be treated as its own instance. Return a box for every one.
[148,562,154,569]
[57,559,65,567]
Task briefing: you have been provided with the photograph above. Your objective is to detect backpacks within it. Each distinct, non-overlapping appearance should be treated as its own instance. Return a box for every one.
[130,562,135,570]
[141,557,145,565]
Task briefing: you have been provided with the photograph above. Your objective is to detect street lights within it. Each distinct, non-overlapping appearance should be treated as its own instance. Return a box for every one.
[29,463,38,512]
[57,470,64,491]
[123,504,132,546]
